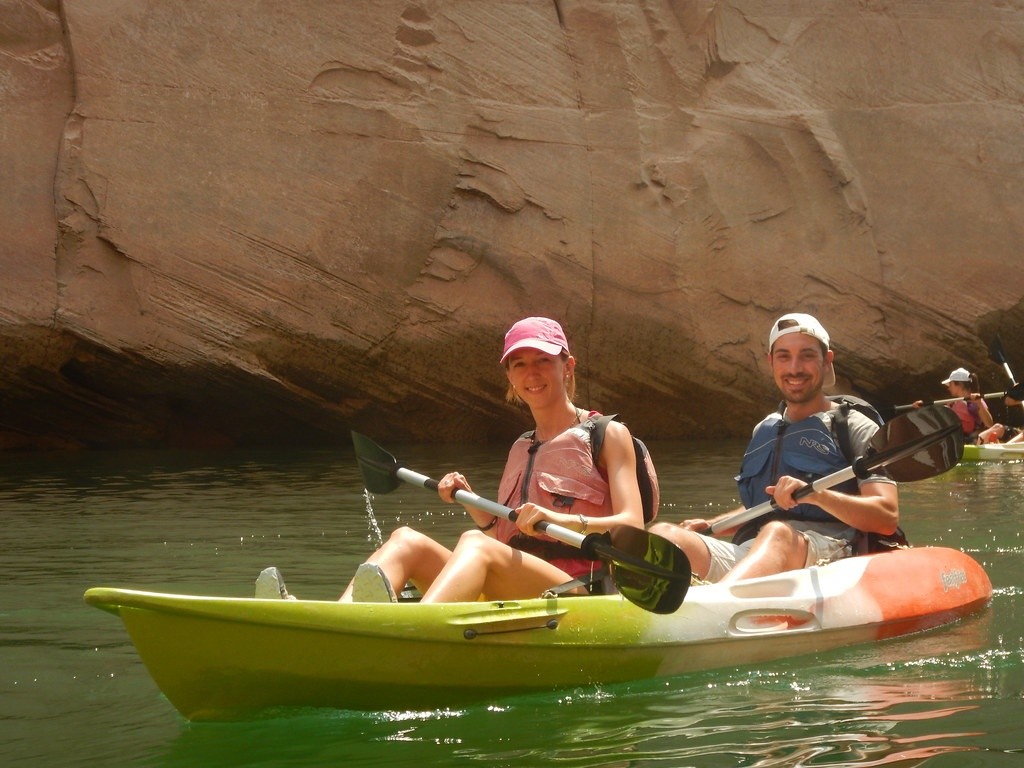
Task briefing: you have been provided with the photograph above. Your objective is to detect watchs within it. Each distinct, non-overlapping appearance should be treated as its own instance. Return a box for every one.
[576,513,588,535]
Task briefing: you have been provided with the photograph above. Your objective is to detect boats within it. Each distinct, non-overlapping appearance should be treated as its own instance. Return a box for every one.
[82,547,990,724]
[961,442,1024,460]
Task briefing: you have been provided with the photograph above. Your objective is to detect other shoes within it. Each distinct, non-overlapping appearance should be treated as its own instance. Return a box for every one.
[352,563,398,603]
[252,567,296,600]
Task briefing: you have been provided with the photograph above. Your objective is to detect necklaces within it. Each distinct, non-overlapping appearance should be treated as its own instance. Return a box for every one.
[527,407,584,455]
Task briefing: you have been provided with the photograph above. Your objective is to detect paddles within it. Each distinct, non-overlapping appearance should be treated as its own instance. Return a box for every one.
[695,404,965,536]
[349,428,693,616]
[880,382,1024,417]
[991,333,1024,409]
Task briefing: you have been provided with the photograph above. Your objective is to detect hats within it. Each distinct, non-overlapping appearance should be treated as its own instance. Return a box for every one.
[769,313,836,387]
[941,367,975,385]
[499,316,570,363]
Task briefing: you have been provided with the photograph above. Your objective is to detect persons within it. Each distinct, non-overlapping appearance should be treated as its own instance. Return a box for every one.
[254,317,645,603]
[912,368,993,445]
[648,312,899,586]
[976,380,1024,445]
